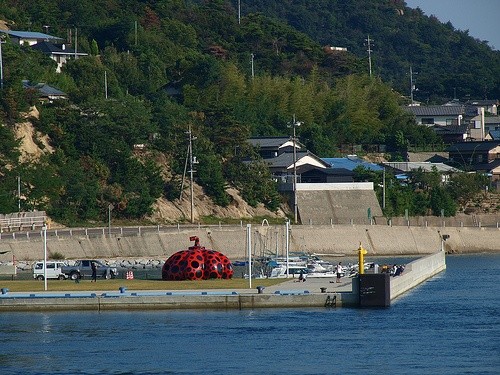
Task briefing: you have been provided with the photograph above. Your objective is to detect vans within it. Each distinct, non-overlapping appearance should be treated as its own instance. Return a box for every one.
[33,262,68,280]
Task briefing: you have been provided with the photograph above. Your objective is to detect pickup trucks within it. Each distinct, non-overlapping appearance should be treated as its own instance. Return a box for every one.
[61,259,119,280]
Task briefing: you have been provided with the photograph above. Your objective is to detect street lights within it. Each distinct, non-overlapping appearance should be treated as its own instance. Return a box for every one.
[184,123,201,224]
[41,223,48,290]
[246,223,251,289]
[406,65,418,108]
[364,34,376,78]
[287,112,306,223]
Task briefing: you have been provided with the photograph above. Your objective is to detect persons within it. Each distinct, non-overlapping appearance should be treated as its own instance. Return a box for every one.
[348,264,357,271]
[294,268,307,283]
[90,262,97,283]
[74,260,80,267]
[37,264,42,269]
[379,263,406,278]
[104,260,113,280]
[335,262,342,283]
[344,269,358,278]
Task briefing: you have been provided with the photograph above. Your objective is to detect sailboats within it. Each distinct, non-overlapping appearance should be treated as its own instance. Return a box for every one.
[232,216,407,279]
